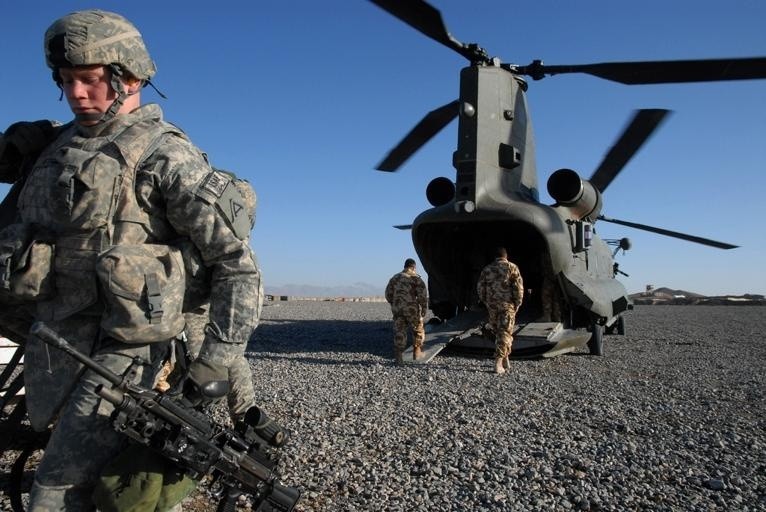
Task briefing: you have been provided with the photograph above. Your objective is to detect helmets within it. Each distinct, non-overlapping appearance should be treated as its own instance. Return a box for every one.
[45,10,155,88]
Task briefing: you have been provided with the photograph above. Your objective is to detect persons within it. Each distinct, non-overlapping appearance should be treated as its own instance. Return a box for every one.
[476,247,524,374]
[182,247,254,415]
[384,259,427,365]
[0,8,266,512]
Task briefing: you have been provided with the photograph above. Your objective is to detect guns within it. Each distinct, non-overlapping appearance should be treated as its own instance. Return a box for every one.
[33,321,306,512]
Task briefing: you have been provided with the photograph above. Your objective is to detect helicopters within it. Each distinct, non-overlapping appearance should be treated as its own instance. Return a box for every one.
[371,0,765,356]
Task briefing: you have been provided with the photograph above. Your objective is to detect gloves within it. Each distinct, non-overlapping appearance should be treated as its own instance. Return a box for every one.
[166,358,229,407]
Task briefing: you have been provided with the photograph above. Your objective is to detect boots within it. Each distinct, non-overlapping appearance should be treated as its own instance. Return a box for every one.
[395,351,403,364]
[494,356,511,373]
[414,347,425,359]
